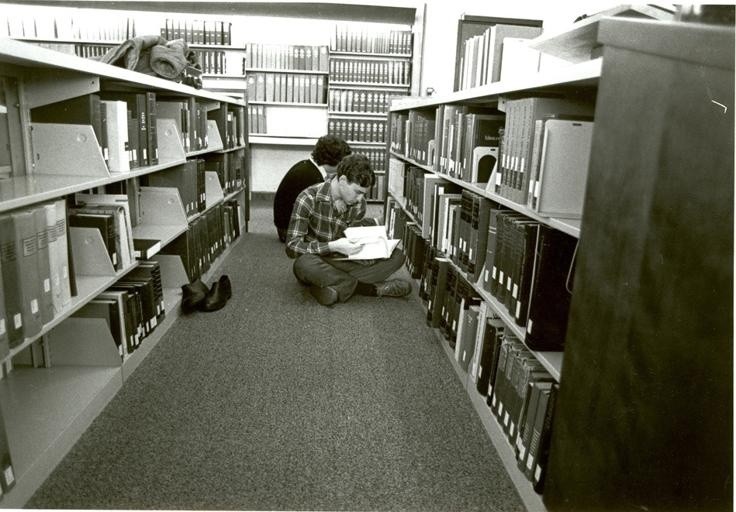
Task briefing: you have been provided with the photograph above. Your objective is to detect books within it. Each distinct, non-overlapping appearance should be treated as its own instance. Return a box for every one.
[344,224,403,262]
[0,66,250,359]
[0,413,16,491]
[1,12,233,73]
[383,5,684,493]
[244,43,267,133]
[267,23,384,203]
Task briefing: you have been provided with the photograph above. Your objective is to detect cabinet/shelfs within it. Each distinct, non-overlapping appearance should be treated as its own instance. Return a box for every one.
[385,6,731,511]
[1,32,249,508]
[247,24,418,213]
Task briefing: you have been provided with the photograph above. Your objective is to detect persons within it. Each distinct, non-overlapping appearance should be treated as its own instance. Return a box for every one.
[274,132,351,249]
[284,151,414,307]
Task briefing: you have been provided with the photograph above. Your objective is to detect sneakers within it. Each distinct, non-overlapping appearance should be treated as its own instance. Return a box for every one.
[180,278,209,320]
[374,278,412,298]
[308,285,338,307]
[204,274,233,311]
